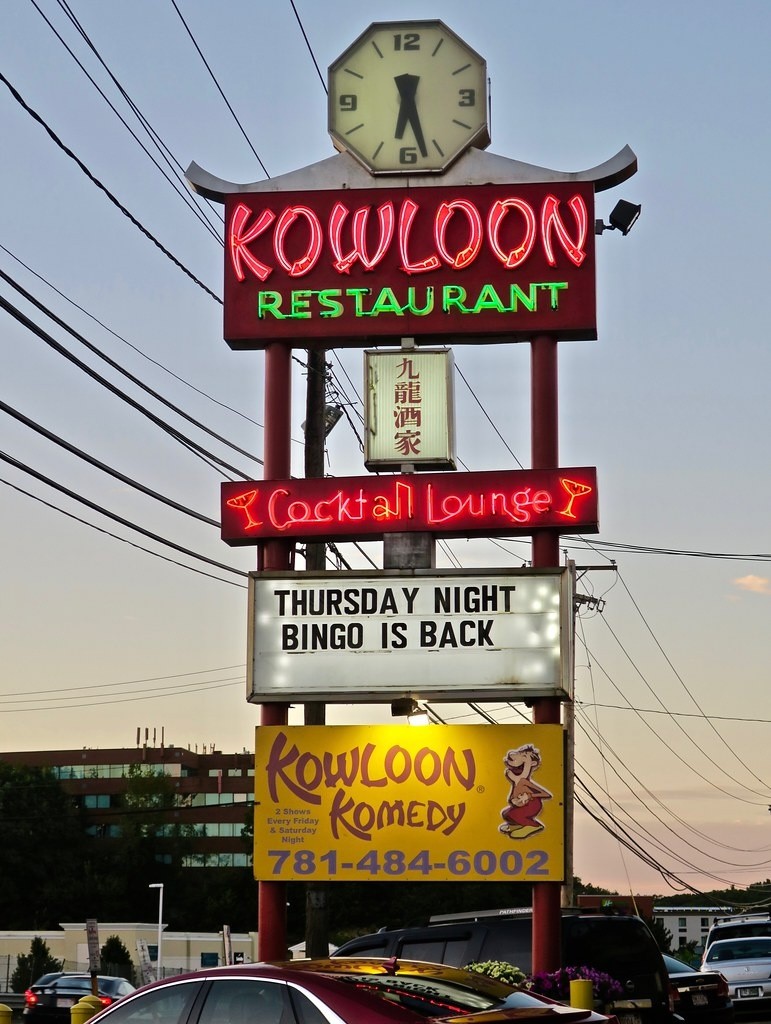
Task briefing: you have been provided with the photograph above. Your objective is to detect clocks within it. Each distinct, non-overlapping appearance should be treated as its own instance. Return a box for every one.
[328,18,491,173]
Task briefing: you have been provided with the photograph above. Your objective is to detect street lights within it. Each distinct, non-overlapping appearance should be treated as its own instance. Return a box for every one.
[149,883,163,981]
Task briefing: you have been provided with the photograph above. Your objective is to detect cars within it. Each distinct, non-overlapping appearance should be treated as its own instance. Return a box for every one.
[22,974,162,1024]
[81,955,620,1024]
[661,952,735,1024]
[701,936,771,1001]
[21,971,91,1007]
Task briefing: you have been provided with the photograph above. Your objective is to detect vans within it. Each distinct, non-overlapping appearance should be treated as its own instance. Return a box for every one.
[329,907,675,1024]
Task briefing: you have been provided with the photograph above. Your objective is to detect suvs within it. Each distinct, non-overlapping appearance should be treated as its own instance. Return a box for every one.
[702,912,771,963]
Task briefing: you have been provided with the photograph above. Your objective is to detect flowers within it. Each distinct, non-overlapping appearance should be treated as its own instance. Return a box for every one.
[546,965,623,993]
[464,960,535,991]
